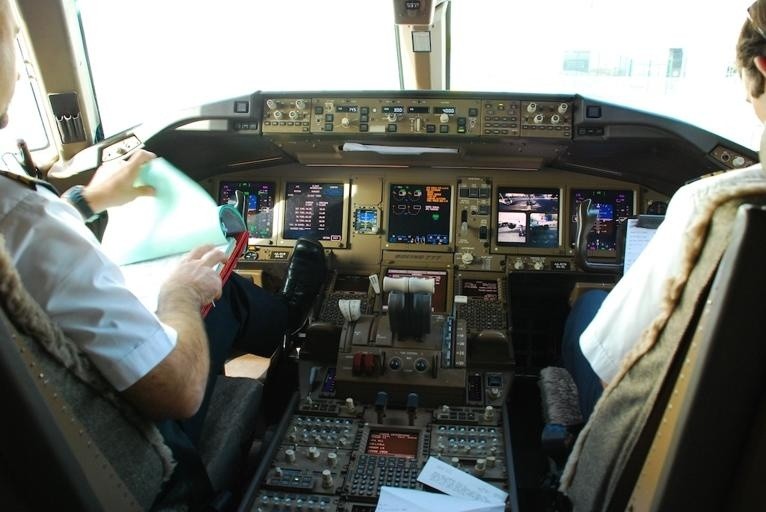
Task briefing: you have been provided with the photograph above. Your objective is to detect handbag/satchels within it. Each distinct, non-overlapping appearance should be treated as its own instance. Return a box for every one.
[58,185,95,220]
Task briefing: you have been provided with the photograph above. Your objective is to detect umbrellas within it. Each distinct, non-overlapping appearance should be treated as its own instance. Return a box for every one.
[283,236,326,314]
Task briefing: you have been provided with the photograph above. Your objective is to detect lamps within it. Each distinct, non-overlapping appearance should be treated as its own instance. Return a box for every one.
[0,235,275,512]
[541,193,766,511]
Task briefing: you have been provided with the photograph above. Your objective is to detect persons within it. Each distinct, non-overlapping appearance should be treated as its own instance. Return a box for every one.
[0,0,330,467]
[557,0,766,441]
